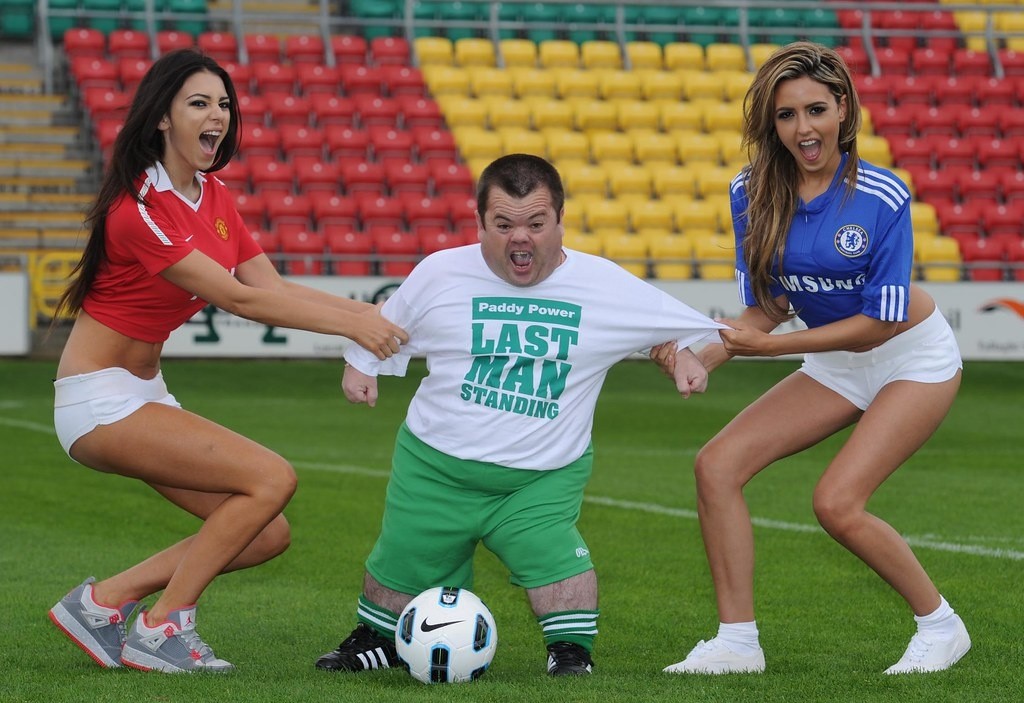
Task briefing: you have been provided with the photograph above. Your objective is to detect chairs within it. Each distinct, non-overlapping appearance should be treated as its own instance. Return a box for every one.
[0,0,1024,284]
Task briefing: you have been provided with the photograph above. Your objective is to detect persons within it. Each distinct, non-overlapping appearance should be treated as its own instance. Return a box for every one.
[651,41,971,678]
[313,151,737,674]
[48,51,412,673]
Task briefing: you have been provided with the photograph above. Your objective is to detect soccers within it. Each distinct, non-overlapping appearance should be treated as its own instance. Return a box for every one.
[394,586,498,684]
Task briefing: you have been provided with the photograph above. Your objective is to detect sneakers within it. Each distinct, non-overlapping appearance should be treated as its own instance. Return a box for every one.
[663,638,765,674]
[49,577,139,668]
[121,605,235,674]
[546,641,595,677]
[883,614,972,674]
[316,622,402,672]
